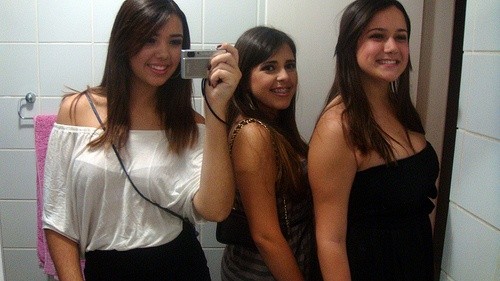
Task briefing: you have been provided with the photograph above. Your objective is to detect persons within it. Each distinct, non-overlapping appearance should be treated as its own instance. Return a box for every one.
[43,0,243,281]
[220,25,310,281]
[308,1,442,281]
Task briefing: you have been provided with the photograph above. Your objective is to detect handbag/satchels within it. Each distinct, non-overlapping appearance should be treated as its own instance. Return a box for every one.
[216,118,291,248]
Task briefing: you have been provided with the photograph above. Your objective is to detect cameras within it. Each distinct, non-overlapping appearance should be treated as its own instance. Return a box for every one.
[181,49,227,79]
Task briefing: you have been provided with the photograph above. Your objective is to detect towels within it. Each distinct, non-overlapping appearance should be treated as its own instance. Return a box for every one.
[35,114,88,281]
